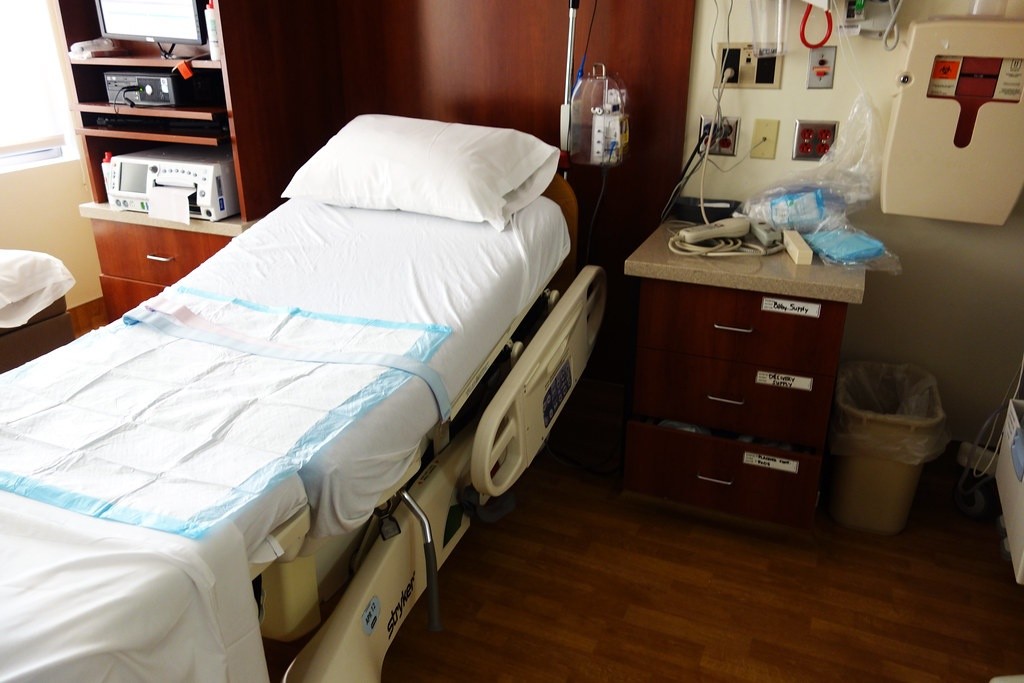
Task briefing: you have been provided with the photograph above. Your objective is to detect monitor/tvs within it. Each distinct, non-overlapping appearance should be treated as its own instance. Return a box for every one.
[95,0,206,47]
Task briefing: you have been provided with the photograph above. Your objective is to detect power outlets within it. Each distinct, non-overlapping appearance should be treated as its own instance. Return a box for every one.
[699,116,738,155]
[714,41,784,90]
[792,119,838,161]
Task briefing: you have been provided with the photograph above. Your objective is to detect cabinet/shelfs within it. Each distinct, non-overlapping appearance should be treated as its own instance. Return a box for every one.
[49,0,325,324]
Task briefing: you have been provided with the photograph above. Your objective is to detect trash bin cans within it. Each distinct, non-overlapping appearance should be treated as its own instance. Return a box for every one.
[832,361,946,538]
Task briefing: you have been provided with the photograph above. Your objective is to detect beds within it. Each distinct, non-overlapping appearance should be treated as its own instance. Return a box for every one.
[0,115,608,682]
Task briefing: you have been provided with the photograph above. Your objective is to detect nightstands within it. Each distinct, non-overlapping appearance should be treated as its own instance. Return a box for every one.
[623,209,866,534]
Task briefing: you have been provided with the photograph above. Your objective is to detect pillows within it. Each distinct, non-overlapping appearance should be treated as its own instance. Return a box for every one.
[281,114,560,232]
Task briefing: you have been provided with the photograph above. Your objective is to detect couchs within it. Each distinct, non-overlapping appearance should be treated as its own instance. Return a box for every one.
[0,249,77,375]
